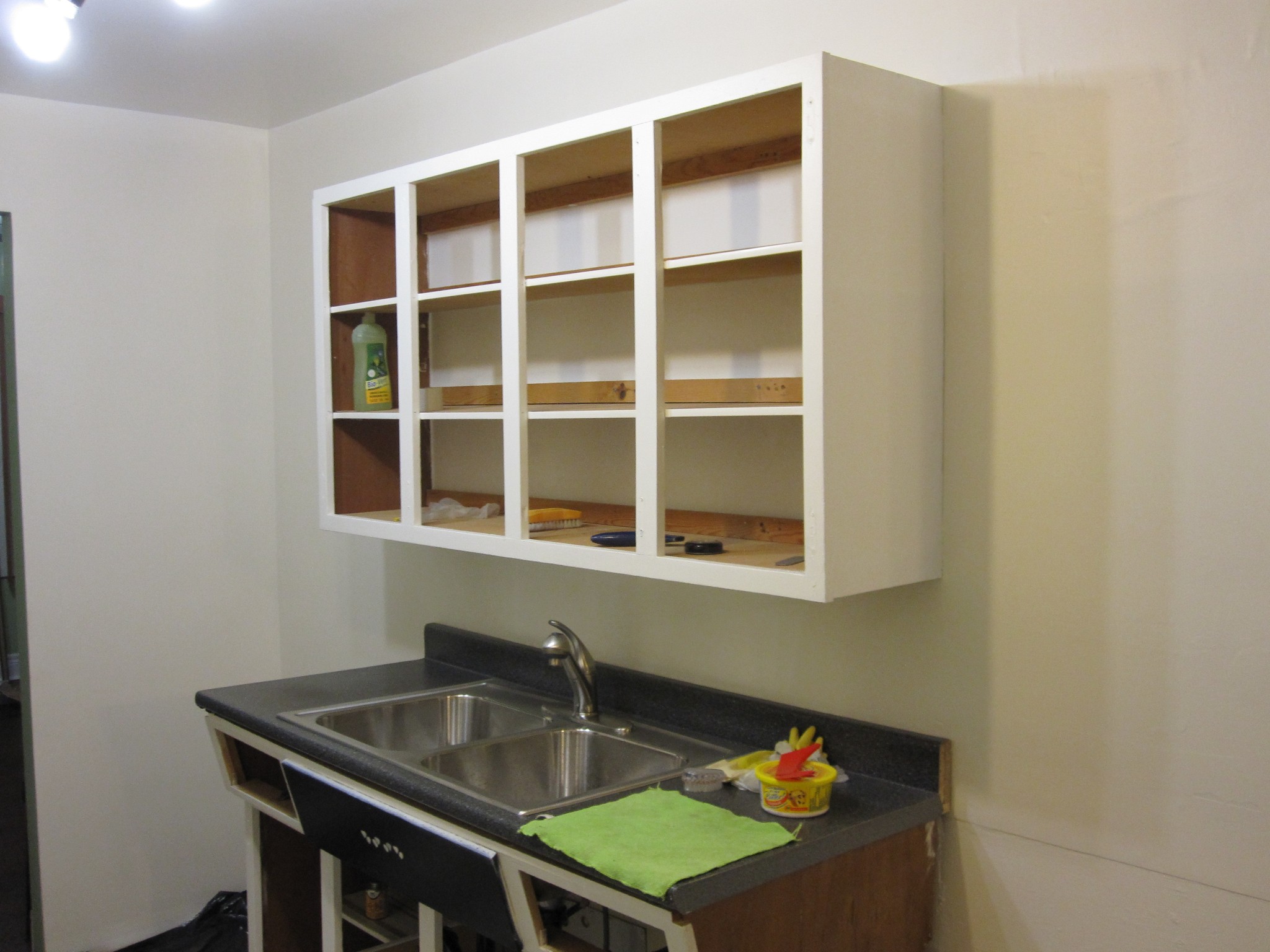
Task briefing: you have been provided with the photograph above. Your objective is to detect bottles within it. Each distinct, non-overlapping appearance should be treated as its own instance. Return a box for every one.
[352,313,393,411]
[366,882,390,919]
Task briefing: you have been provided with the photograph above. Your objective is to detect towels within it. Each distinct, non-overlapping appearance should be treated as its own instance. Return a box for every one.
[517,780,806,903]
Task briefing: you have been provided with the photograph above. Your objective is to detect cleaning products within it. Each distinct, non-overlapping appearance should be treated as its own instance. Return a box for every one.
[350,312,395,412]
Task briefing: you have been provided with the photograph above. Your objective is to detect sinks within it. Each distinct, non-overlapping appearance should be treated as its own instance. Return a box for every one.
[276,676,569,768]
[415,717,745,817]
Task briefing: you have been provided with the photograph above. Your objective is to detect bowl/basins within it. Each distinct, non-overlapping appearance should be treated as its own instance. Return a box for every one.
[754,760,837,818]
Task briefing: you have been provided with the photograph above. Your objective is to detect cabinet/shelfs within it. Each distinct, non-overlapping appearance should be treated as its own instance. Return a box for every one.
[239,805,940,952]
[314,45,947,603]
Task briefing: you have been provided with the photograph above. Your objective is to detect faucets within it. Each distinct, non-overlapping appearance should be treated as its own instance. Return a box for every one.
[539,616,601,724]
